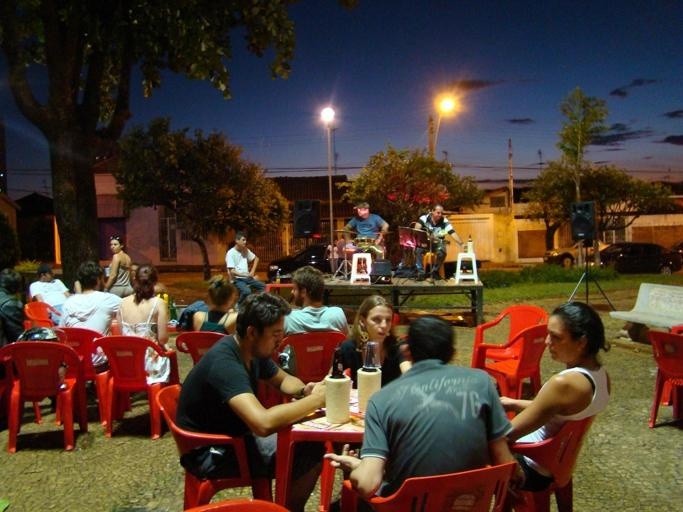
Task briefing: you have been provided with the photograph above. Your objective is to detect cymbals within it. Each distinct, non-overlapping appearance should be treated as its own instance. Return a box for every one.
[332,229,358,235]
[373,230,393,235]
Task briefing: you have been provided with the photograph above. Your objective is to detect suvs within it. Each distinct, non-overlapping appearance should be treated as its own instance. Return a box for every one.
[266,245,334,279]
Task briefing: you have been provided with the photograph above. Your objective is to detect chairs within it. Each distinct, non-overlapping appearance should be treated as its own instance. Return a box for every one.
[49,321,103,394]
[154,384,274,500]
[661,326,683,405]
[285,326,355,385]
[94,336,180,444]
[174,324,229,368]
[646,331,683,428]
[3,342,85,456]
[339,459,513,508]
[19,304,57,334]
[470,303,552,397]
[509,418,593,510]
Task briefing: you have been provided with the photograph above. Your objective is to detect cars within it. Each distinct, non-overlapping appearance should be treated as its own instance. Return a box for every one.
[543,238,612,267]
[586,242,681,276]
[671,238,683,256]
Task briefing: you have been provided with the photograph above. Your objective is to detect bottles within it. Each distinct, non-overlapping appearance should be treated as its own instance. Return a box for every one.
[275,270,281,284]
[464,245,468,253]
[330,348,346,422]
[468,234,474,242]
[103,265,111,277]
[361,343,377,411]
[462,266,467,274]
[156,292,178,321]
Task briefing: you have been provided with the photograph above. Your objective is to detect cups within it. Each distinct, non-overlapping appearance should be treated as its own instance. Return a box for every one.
[325,375,351,424]
[357,368,382,414]
[362,340,382,370]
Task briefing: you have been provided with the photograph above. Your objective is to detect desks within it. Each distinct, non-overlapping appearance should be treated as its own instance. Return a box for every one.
[273,380,508,510]
[265,283,296,298]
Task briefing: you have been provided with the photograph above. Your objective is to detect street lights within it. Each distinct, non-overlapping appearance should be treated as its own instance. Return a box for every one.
[319,106,335,245]
[430,93,456,159]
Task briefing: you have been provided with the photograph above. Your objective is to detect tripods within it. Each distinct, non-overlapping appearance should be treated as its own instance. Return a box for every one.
[567,249,617,310]
[333,244,352,280]
[402,240,432,285]
[419,239,449,286]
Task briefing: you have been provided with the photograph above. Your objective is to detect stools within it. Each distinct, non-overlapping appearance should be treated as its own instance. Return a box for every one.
[350,253,371,285]
[423,252,445,278]
[455,253,478,285]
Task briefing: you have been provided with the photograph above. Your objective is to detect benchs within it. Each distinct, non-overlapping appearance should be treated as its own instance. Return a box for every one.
[609,283,683,340]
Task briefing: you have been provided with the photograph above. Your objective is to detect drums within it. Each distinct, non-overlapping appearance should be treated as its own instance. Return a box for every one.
[354,237,375,246]
[354,246,382,274]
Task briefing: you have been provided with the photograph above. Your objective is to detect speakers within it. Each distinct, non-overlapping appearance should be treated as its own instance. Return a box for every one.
[569,200,597,243]
[293,201,320,239]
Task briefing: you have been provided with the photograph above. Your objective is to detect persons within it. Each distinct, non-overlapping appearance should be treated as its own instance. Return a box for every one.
[342,203,389,281]
[414,204,467,284]
[1,232,170,413]
[193,274,239,335]
[280,266,350,370]
[332,295,408,389]
[499,302,613,491]
[327,314,515,512]
[225,231,264,309]
[176,292,327,512]
